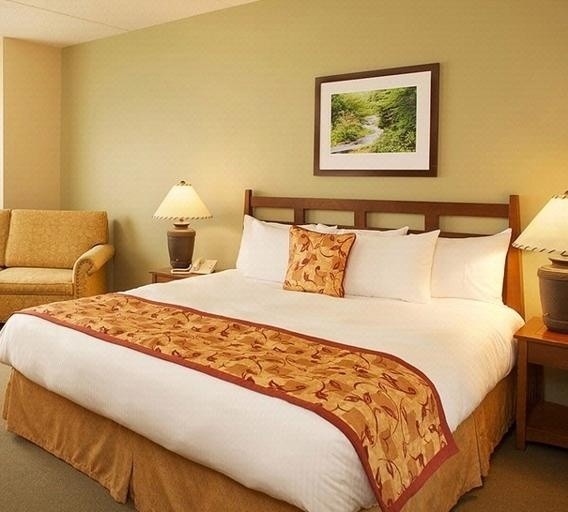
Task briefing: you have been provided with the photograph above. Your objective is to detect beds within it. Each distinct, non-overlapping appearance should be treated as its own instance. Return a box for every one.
[0,191,525,512]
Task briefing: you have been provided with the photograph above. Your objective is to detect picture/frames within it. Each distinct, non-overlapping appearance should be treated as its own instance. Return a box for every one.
[314,63,440,177]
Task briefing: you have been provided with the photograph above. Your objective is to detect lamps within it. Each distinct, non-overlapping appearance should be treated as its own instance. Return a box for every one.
[512,191,568,332]
[152,180,213,269]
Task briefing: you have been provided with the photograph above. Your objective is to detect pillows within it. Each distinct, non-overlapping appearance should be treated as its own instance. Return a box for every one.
[236,214,513,306]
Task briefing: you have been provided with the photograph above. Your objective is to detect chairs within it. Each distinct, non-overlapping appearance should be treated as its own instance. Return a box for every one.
[0,208,115,324]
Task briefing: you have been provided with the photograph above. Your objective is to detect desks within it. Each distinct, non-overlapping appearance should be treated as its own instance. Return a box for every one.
[513,316,568,451]
[149,267,216,284]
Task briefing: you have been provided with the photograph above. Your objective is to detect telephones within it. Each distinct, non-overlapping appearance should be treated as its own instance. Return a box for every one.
[189,258,218,275]
